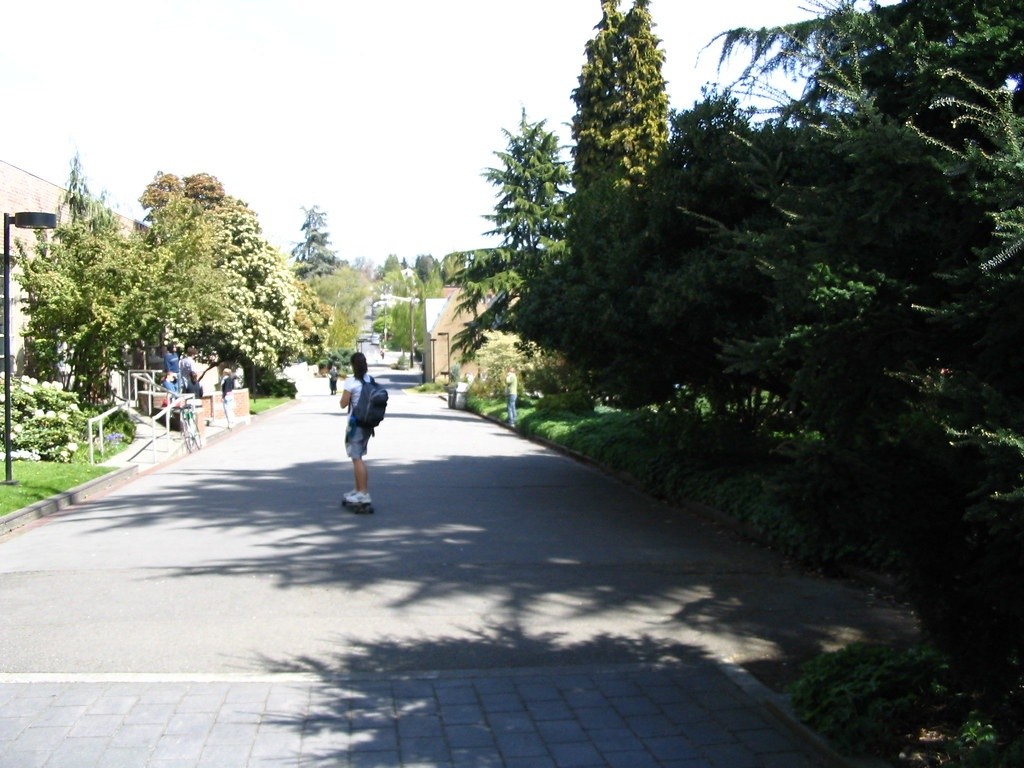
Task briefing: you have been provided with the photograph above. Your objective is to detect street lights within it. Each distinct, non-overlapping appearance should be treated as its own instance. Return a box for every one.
[437,332,451,386]
[0,212,57,485]
[429,338,436,383]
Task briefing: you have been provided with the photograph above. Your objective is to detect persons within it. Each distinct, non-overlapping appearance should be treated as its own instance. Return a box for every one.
[161,343,339,430]
[340,352,374,504]
[504,368,518,426]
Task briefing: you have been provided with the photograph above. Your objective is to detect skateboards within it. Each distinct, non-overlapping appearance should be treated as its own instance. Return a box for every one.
[342,498,374,514]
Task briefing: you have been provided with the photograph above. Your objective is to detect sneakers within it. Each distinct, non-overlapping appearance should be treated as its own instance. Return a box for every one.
[346,492,372,504]
[343,488,359,499]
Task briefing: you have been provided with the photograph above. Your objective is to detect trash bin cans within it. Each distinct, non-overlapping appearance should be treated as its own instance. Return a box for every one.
[447,382,470,410]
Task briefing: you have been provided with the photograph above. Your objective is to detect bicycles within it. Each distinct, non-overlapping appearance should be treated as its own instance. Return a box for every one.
[170,396,203,455]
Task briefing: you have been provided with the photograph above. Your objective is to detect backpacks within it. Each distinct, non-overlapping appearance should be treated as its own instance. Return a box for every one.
[347,375,389,437]
